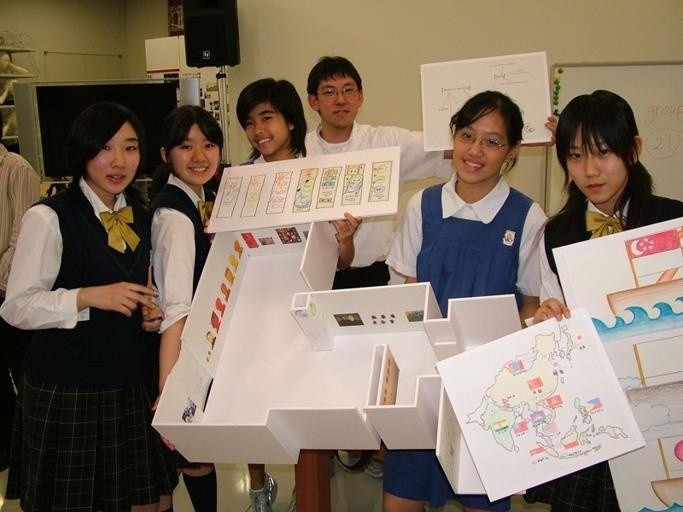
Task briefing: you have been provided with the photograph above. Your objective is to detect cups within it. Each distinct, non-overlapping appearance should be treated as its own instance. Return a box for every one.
[318,85,359,97]
[455,127,509,150]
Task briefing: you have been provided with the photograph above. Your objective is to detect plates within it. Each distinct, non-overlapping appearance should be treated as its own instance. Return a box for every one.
[0,46,39,142]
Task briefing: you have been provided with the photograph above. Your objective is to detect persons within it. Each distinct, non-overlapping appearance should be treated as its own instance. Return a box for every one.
[233,75,362,511]
[0,51,29,153]
[0,139,44,480]
[527,89,683,511]
[145,102,230,511]
[287,57,566,263]
[0,102,167,511]
[367,91,550,511]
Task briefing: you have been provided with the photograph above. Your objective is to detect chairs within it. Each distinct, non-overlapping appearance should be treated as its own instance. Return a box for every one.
[355,457,384,478]
[249,472,278,512]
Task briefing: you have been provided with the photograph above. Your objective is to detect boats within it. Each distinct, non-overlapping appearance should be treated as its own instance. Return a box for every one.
[182,0,240,67]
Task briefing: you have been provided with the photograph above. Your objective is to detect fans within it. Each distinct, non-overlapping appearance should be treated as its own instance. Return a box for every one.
[544,60,683,220]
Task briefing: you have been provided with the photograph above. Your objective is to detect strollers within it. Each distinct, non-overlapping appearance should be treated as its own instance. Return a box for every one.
[583,210,627,239]
[99,206,142,255]
[197,199,213,225]
